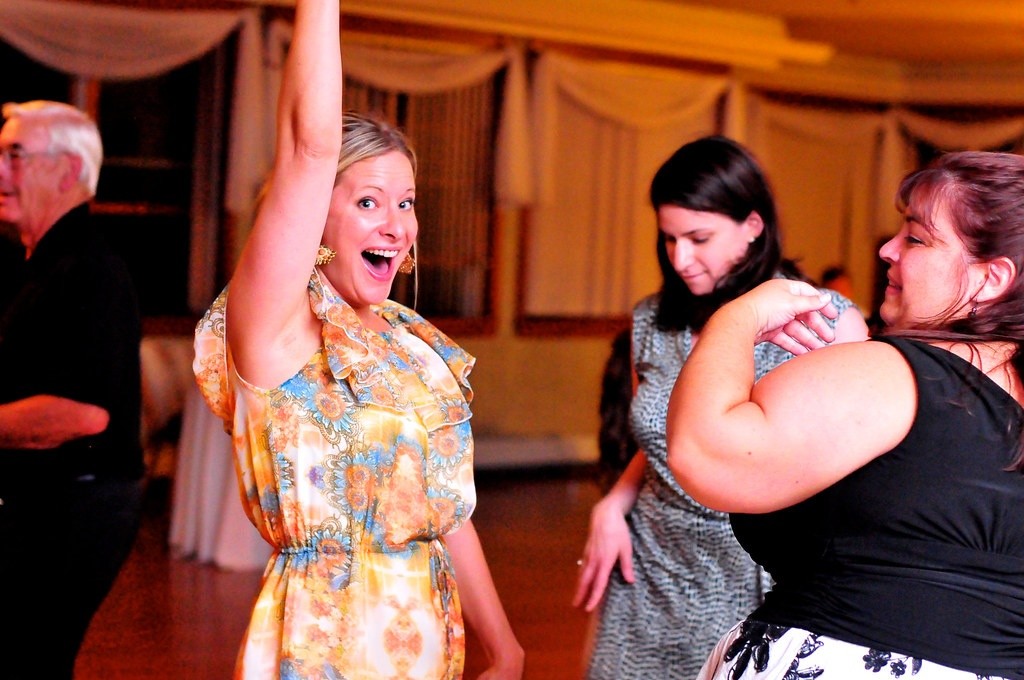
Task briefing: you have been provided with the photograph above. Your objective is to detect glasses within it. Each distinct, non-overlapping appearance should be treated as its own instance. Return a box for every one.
[0,141,67,171]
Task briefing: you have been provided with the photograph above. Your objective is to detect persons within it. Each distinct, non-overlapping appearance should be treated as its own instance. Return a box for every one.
[571,135,870,680]
[0,100,143,680]
[192,0,525,680]
[667,151,1024,680]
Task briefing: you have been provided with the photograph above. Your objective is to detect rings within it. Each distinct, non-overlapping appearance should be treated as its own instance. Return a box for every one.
[577,560,584,566]
